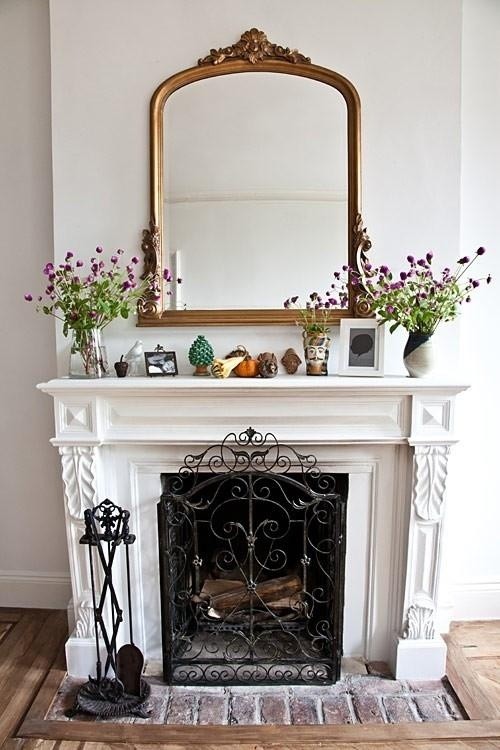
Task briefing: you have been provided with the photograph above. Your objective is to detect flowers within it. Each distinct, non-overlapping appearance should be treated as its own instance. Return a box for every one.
[24,244,171,371]
[331,244,492,334]
[283,292,348,331]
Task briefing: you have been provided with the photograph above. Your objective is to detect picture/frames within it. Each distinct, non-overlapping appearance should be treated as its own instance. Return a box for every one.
[338,316,386,378]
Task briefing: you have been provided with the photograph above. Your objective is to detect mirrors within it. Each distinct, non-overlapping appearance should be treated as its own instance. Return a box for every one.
[136,28,376,329]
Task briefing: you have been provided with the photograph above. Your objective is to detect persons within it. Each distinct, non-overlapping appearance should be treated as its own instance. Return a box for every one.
[149,360,176,372]
[308,344,327,374]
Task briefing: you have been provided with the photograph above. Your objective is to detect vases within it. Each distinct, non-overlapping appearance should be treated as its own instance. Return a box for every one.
[301,333,332,376]
[403,332,437,379]
[67,328,110,380]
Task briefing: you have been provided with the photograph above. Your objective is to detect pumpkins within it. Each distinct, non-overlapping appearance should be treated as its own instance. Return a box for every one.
[233,359,260,377]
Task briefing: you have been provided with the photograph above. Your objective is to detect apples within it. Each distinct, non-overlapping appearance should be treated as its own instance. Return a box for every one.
[114,362,128,377]
